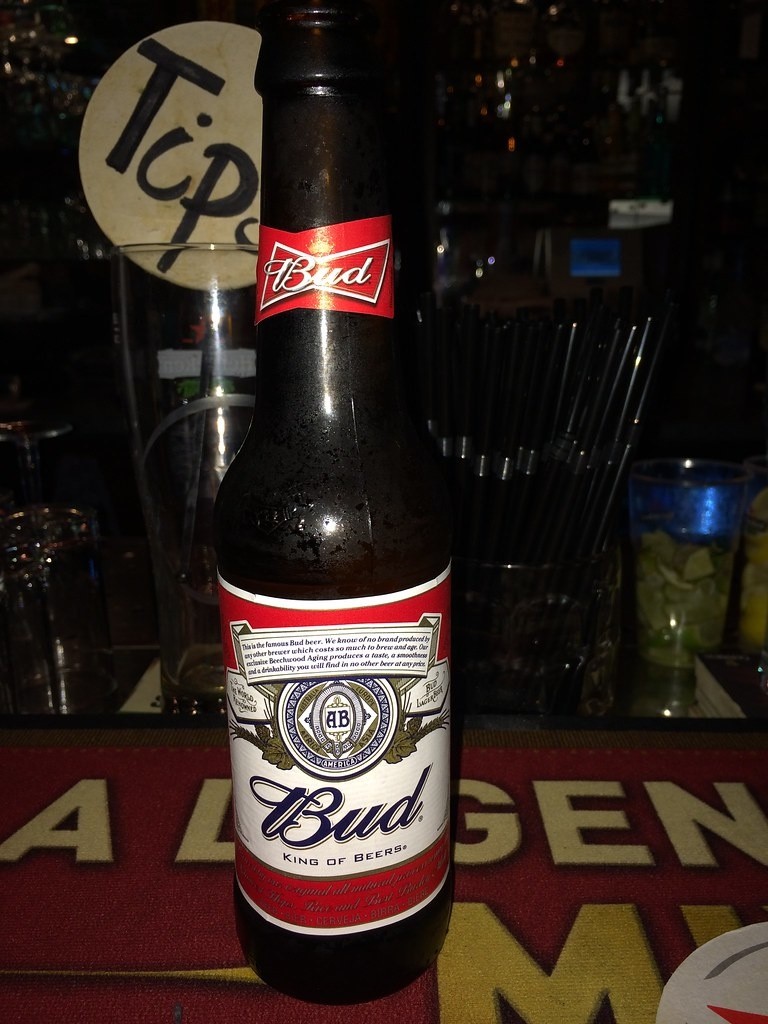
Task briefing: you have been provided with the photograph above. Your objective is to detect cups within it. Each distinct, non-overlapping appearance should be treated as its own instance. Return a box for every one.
[734,446,768,660]
[0,503,121,719]
[118,238,262,730]
[623,454,753,668]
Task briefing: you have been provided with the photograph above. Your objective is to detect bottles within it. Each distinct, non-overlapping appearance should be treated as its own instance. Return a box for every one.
[214,1,455,1008]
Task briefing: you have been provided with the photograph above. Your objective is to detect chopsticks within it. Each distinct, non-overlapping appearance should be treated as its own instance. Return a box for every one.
[427,288,676,715]
